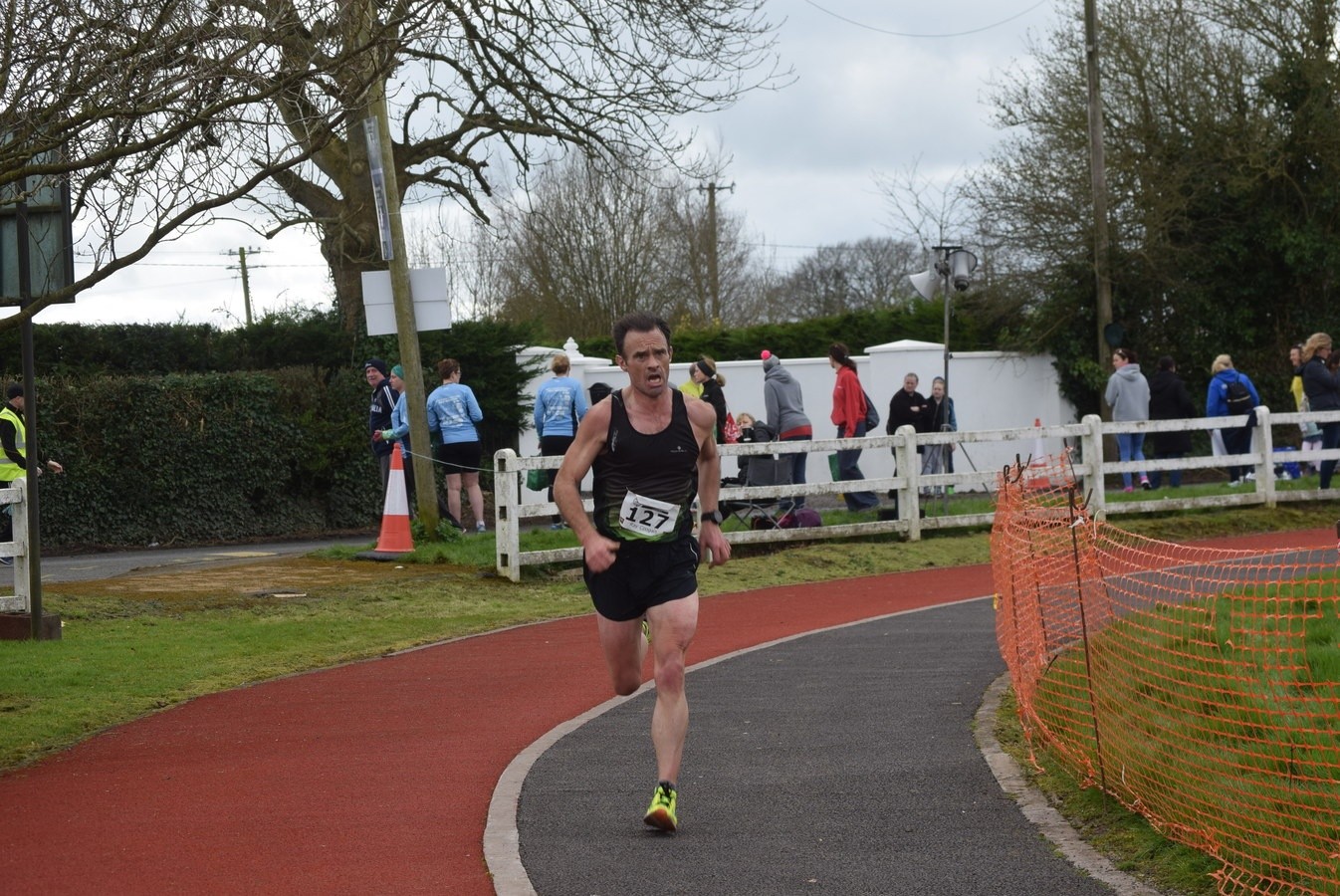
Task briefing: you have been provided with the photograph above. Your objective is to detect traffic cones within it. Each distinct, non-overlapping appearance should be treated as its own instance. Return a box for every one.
[354,443,417,564]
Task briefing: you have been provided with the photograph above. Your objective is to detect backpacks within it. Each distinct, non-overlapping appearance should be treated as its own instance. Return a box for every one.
[1215,373,1253,415]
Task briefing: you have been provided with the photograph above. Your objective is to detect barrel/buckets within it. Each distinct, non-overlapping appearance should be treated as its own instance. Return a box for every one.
[1273,445,1300,479]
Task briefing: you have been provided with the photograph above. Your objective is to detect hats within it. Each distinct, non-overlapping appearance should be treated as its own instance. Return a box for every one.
[7,383,24,398]
[762,350,780,373]
[393,364,404,380]
[365,360,386,378]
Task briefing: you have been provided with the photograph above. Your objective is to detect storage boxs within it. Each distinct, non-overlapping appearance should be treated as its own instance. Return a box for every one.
[1274,445,1301,480]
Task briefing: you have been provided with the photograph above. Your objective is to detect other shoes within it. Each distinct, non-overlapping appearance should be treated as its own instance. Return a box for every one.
[858,501,880,512]
[889,492,897,499]
[935,489,942,497]
[550,524,563,530]
[1,558,14,565]
[477,525,486,533]
[947,487,954,495]
[924,487,931,496]
[1227,481,1240,485]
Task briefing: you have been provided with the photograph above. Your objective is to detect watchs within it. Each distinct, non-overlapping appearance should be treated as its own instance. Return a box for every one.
[701,510,723,526]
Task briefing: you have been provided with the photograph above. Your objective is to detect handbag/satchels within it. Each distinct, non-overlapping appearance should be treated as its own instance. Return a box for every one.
[527,452,549,491]
[864,393,879,432]
[828,453,842,482]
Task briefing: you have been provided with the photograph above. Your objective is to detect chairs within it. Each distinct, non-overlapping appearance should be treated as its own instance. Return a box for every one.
[1185,441,1230,480]
[725,456,797,529]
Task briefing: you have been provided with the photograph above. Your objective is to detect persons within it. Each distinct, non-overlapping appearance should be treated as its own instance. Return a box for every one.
[828,342,880,513]
[678,355,726,445]
[761,349,813,510]
[365,360,486,536]
[553,311,731,832]
[1290,333,1340,491]
[0,384,63,566]
[886,373,957,499]
[1105,347,1152,494]
[1206,354,1260,488]
[1148,357,1198,489]
[691,414,778,535]
[534,356,588,531]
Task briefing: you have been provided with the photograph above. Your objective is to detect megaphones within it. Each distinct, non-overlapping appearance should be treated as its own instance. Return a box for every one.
[907,261,948,301]
[946,251,978,292]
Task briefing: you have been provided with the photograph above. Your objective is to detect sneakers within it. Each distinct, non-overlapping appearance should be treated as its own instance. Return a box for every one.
[643,781,677,832]
[642,616,652,644]
[1141,476,1151,489]
[1124,486,1133,493]
